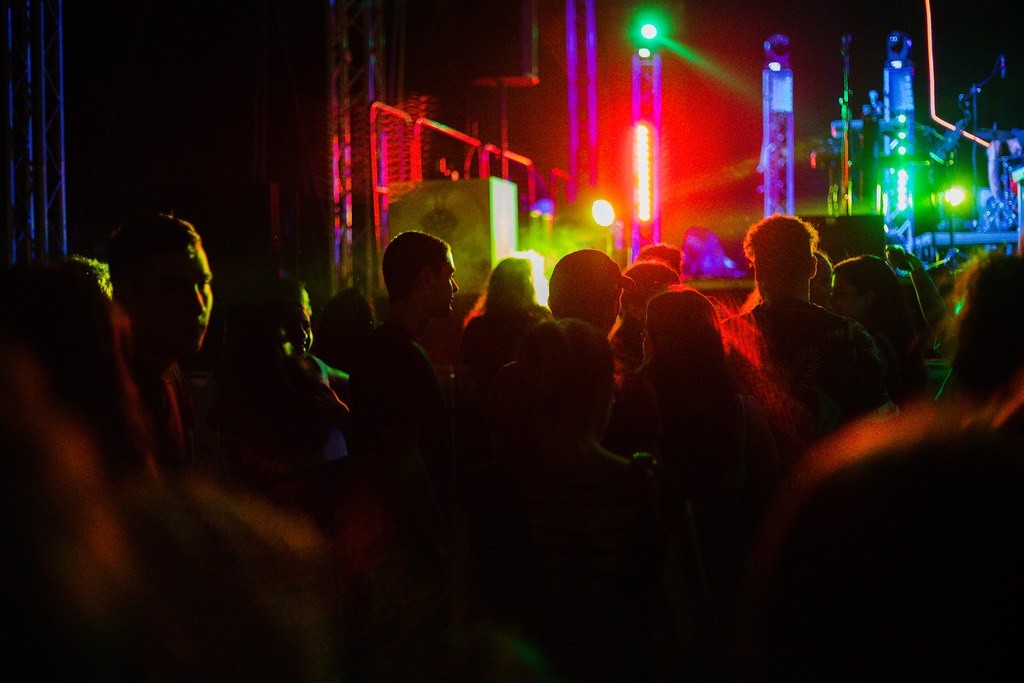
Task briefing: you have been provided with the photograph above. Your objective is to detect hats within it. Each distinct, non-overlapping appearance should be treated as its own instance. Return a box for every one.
[549,250,634,301]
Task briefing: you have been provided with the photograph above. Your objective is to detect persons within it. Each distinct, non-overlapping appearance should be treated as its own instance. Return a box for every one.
[0,212,1024,683]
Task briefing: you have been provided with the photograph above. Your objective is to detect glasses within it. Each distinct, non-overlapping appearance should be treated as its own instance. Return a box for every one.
[823,293,855,308]
